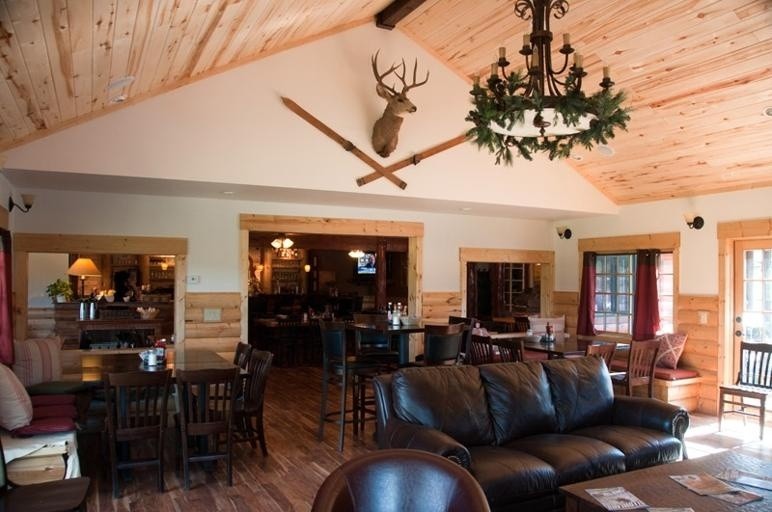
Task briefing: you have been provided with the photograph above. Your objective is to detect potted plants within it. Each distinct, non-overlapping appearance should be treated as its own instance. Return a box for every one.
[89,292,99,320]
[45,279,76,303]
[76,294,89,321]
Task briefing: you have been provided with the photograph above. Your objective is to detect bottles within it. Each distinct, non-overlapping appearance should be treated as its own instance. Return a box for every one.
[545,321,554,342]
[526,329,533,336]
[387,301,407,324]
[148,348,157,365]
[150,269,169,278]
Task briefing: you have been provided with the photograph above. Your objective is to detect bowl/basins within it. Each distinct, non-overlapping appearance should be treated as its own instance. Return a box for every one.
[523,336,541,343]
[400,316,420,326]
[137,309,159,320]
[142,294,170,303]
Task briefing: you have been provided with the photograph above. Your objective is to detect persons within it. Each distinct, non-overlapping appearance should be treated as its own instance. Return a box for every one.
[361,255,376,269]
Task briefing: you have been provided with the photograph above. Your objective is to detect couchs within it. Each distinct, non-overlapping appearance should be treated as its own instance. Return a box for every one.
[370,352,691,512]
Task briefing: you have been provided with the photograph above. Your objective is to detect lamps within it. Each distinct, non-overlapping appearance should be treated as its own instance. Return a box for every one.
[64,255,102,299]
[7,192,40,215]
[465,0,638,168]
[555,224,573,241]
[681,210,703,230]
[270,231,294,250]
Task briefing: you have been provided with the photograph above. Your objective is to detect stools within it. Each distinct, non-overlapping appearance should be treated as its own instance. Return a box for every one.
[317,319,379,452]
[354,313,396,433]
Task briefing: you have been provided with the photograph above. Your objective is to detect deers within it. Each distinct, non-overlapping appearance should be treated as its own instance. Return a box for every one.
[370,47,430,159]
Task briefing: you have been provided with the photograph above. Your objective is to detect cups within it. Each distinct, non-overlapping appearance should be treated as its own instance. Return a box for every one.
[90,302,98,320]
[124,297,130,302]
[80,302,87,319]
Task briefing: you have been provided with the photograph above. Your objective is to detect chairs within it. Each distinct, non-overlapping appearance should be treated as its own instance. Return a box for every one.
[102,368,173,499]
[612,341,662,401]
[717,340,772,440]
[249,299,617,394]
[186,341,252,446]
[310,447,492,511]
[199,348,276,458]
[174,365,241,490]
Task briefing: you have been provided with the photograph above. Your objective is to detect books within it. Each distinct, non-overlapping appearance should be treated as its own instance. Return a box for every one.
[583,465,771,512]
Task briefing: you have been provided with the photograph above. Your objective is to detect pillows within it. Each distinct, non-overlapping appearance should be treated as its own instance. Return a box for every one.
[648,331,688,371]
[0,361,34,433]
[10,334,68,387]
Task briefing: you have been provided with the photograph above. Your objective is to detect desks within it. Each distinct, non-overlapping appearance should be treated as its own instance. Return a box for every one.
[80,346,237,483]
